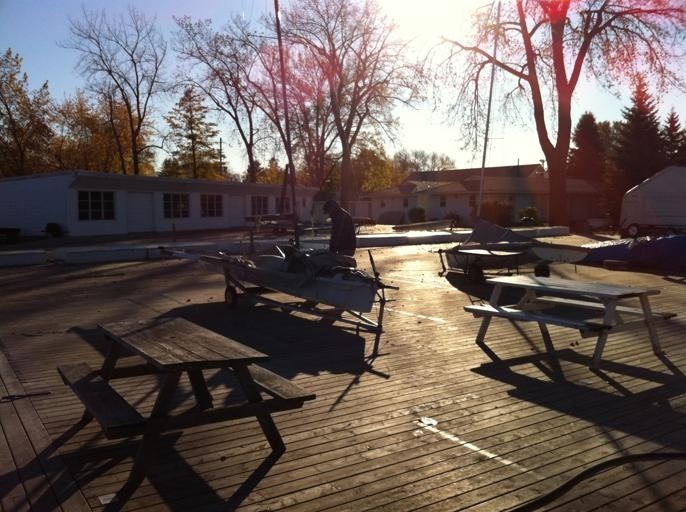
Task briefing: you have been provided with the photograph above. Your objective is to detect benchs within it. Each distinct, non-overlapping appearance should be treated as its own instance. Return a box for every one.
[464,295,676,370]
[55,363,315,486]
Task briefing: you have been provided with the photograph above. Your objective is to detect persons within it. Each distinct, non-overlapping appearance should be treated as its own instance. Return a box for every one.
[322,200,356,257]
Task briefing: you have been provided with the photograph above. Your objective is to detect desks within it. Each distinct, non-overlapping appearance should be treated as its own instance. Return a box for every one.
[475,275,661,370]
[457,249,526,282]
[81,316,285,485]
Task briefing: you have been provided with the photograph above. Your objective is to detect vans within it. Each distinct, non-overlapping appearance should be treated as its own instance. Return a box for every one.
[619,166,686,239]
